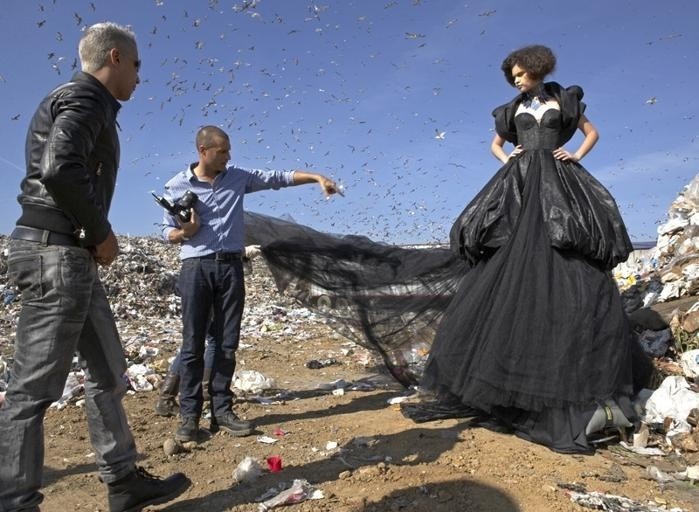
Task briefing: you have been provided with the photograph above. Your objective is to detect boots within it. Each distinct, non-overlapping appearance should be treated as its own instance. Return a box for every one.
[156,373,179,416]
[202,368,211,399]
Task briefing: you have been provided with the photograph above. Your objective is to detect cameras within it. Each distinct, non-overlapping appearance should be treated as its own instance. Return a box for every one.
[151,188,198,222]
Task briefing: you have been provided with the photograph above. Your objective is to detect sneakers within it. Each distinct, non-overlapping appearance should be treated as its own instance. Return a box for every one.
[211,413,253,436]
[177,414,199,441]
[108,467,191,512]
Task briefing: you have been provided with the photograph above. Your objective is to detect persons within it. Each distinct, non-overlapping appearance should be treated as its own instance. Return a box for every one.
[162,125,345,442]
[421,44,671,456]
[155,244,262,418]
[0,21,191,512]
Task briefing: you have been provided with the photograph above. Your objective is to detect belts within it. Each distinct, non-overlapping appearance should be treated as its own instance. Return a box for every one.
[202,253,241,261]
[11,227,91,248]
